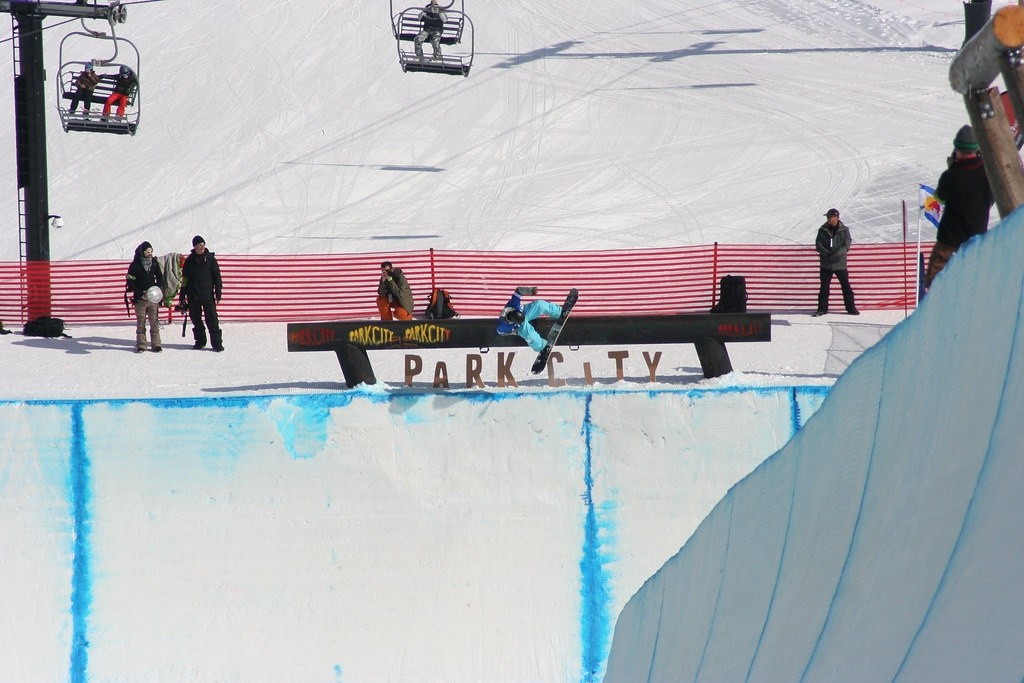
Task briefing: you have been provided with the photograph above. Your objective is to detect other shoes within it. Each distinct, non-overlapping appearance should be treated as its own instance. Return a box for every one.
[154,346,163,352]
[848,308,859,314]
[560,310,569,318]
[813,310,826,317]
[137,347,144,353]
[542,345,552,353]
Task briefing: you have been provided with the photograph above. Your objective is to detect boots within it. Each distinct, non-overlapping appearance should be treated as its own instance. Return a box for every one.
[192,326,207,349]
[209,328,223,352]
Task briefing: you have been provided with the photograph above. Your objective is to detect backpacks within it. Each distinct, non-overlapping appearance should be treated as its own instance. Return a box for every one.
[425,289,454,319]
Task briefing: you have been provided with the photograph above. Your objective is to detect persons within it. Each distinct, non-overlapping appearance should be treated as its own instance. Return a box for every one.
[179,235,224,351]
[69,62,100,121]
[124,241,165,353]
[811,209,860,317]
[925,124,997,288]
[414,0,448,63]
[100,66,137,123]
[377,262,414,320]
[496,286,568,359]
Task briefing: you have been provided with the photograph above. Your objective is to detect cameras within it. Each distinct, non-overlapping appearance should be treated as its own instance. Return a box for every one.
[386,269,393,276]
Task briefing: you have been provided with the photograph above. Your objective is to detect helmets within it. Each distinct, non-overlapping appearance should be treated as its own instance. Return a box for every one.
[511,309,524,325]
[120,67,131,78]
[85,62,93,73]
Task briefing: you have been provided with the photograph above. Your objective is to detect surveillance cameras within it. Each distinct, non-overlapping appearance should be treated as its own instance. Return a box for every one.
[52,218,64,228]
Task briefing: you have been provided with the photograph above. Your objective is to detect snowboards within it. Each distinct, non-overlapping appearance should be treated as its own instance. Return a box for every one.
[531,287,579,374]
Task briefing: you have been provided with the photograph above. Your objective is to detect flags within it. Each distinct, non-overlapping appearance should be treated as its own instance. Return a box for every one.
[919,184,945,228]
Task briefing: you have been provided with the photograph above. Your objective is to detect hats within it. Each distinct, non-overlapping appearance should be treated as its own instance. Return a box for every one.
[192,236,205,246]
[825,209,839,217]
[953,123,979,151]
[141,241,153,252]
